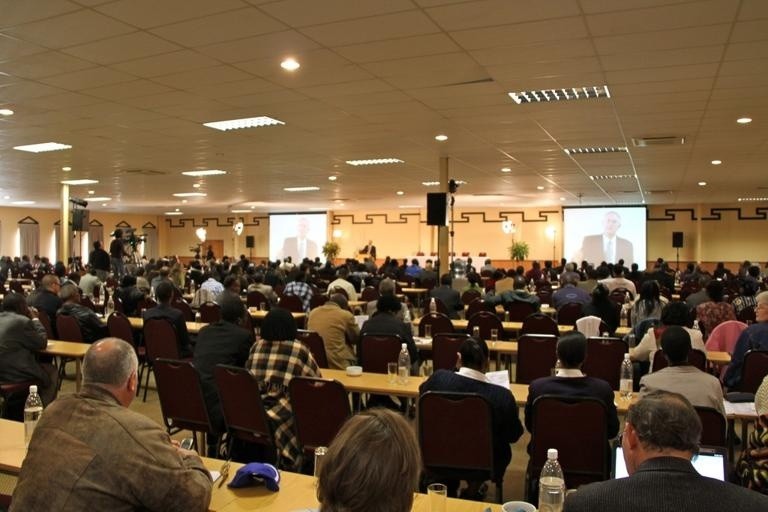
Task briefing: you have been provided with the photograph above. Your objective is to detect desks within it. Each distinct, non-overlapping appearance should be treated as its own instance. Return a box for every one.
[0,418,504,512]
[319,367,757,422]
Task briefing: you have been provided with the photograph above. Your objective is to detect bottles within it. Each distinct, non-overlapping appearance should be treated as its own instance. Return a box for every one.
[7,268,12,280]
[620,304,627,329]
[107,295,114,318]
[602,332,610,345]
[624,292,630,303]
[538,449,565,512]
[149,286,154,300]
[24,385,43,449]
[620,353,633,400]
[398,343,410,385]
[190,279,195,297]
[429,298,436,318]
[692,320,700,332]
[31,280,36,291]
[99,286,105,305]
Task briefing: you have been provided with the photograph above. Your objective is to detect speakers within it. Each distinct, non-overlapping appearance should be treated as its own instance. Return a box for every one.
[74,208,91,231]
[244,235,254,250]
[425,192,448,228]
[671,230,685,248]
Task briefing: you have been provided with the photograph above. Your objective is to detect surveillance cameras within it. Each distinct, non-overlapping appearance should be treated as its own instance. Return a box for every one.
[448,176,458,194]
[69,194,88,208]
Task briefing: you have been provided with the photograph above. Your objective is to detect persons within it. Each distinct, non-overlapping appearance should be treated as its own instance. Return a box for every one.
[5,332,216,512]
[0,228,766,417]
[313,402,425,512]
[280,219,320,268]
[217,307,326,472]
[639,325,729,444]
[188,299,258,456]
[578,209,635,268]
[561,389,768,512]
[523,329,621,481]
[419,335,524,504]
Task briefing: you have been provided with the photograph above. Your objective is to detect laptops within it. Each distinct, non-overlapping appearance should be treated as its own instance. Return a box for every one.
[612,445,730,486]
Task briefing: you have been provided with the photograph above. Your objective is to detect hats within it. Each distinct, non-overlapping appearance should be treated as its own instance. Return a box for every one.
[228,462,280,491]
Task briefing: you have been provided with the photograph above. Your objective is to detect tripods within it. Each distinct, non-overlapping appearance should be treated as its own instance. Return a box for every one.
[127,250,143,268]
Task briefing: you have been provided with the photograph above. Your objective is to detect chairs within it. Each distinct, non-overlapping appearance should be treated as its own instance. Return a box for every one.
[213,364,280,469]
[619,405,729,457]
[0,381,39,422]
[522,395,619,510]
[418,391,501,503]
[152,358,227,459]
[286,376,352,472]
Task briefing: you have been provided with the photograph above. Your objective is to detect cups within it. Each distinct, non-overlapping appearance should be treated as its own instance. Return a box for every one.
[260,303,266,314]
[502,501,536,512]
[425,325,431,339]
[427,483,447,512]
[473,326,479,337]
[195,313,201,329]
[314,447,329,486]
[629,334,636,348]
[491,329,497,347]
[388,363,397,384]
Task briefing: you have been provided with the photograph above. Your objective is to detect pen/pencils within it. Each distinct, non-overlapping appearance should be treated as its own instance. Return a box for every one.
[218,473,228,488]
